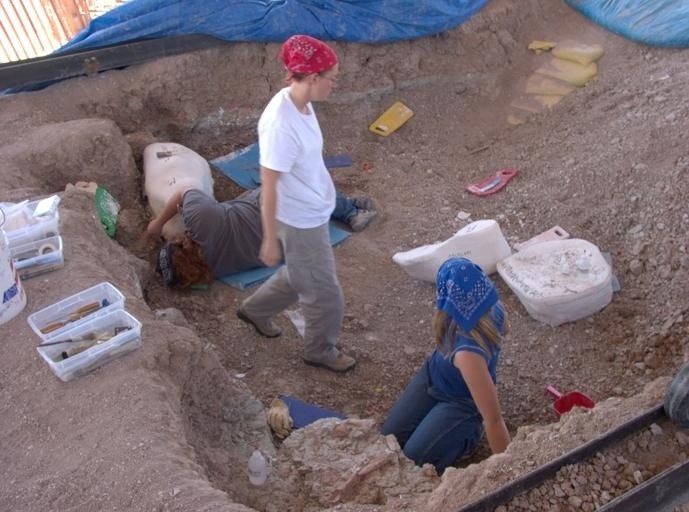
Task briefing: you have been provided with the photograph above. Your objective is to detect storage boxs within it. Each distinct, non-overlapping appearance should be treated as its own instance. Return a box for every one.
[0,197,143,382]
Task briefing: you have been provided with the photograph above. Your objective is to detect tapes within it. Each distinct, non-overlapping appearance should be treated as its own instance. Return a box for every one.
[39,245,58,256]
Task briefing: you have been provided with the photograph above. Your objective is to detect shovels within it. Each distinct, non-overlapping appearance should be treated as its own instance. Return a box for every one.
[545,386,596,417]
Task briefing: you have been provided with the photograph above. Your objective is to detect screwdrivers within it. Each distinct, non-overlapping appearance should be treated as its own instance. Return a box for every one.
[38,301,109,359]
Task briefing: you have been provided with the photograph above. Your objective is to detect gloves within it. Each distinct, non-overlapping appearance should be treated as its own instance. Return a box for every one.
[268,397,295,439]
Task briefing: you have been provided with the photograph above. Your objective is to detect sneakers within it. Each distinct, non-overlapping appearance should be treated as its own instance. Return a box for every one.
[353,196,372,210]
[302,347,356,373]
[348,209,378,233]
[236,304,282,338]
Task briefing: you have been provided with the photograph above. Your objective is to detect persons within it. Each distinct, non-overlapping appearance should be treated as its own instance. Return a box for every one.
[383,255,509,474]
[147,188,379,291]
[237,35,356,374]
